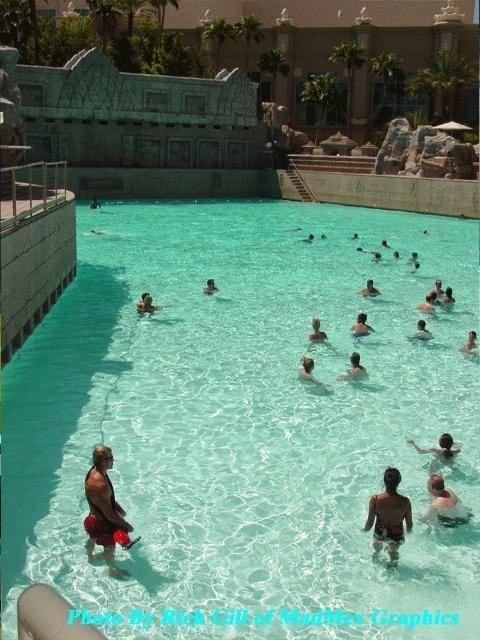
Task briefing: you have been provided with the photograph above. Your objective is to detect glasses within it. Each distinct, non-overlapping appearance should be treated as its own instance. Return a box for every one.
[102,456,114,461]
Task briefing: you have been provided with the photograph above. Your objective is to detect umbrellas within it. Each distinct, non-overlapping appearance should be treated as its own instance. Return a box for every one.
[431,120,474,155]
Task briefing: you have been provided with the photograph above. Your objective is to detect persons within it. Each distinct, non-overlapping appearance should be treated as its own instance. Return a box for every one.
[320,235,330,239]
[362,466,413,571]
[297,357,319,378]
[351,233,422,274]
[406,433,464,460]
[84,443,134,582]
[90,197,101,209]
[360,279,381,296]
[414,318,430,336]
[344,352,363,374]
[421,474,472,530]
[349,314,373,332]
[304,234,314,245]
[308,318,328,340]
[461,329,479,357]
[202,278,220,296]
[137,293,158,313]
[418,278,456,312]
